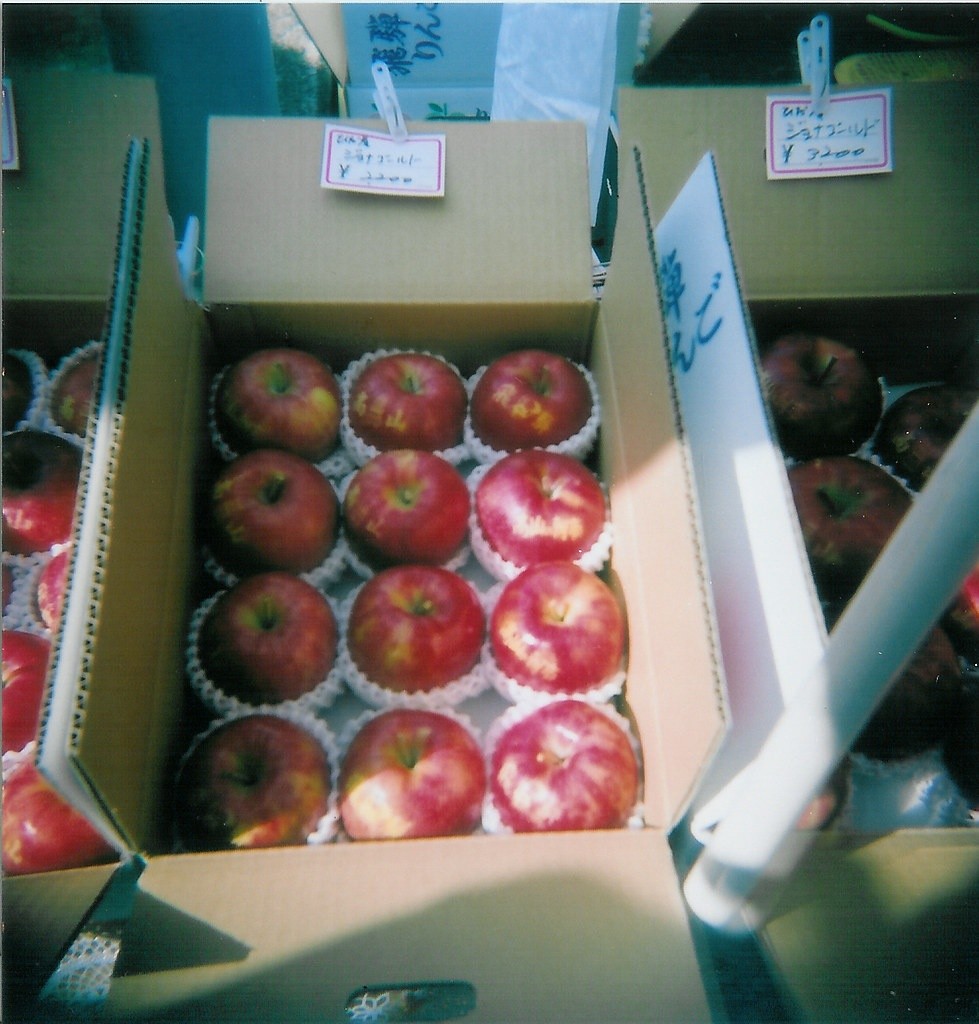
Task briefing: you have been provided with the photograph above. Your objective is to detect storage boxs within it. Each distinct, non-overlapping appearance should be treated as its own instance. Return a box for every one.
[29,115,731,1024]
[610,85,979,1024]
[0,61,159,1024]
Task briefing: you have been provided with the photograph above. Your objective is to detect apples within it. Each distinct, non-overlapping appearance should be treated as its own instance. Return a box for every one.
[763,328,979,814]
[0,343,122,877]
[173,345,637,849]
